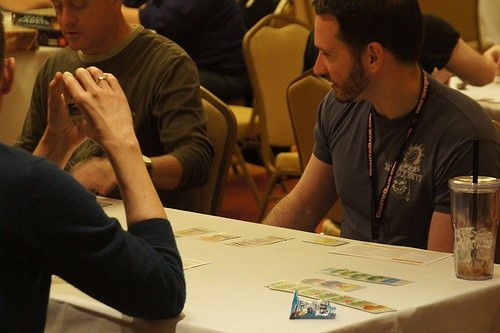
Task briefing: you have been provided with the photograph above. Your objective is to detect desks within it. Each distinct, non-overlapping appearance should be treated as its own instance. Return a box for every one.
[45,196,500,333]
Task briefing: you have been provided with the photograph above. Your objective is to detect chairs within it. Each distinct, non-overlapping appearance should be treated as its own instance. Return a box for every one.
[181,0,335,216]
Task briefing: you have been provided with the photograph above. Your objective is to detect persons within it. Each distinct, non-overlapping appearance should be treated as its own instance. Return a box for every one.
[259,0,500,264]
[0,9,187,333]
[123,0,500,167]
[14,0,217,211]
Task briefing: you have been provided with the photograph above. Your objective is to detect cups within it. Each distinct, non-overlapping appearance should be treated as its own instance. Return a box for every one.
[447,175,500,280]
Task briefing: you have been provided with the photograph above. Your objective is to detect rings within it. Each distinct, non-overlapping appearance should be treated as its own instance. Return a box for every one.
[96,76,106,84]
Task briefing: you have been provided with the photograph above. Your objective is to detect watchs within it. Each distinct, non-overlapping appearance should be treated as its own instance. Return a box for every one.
[141,155,152,176]
